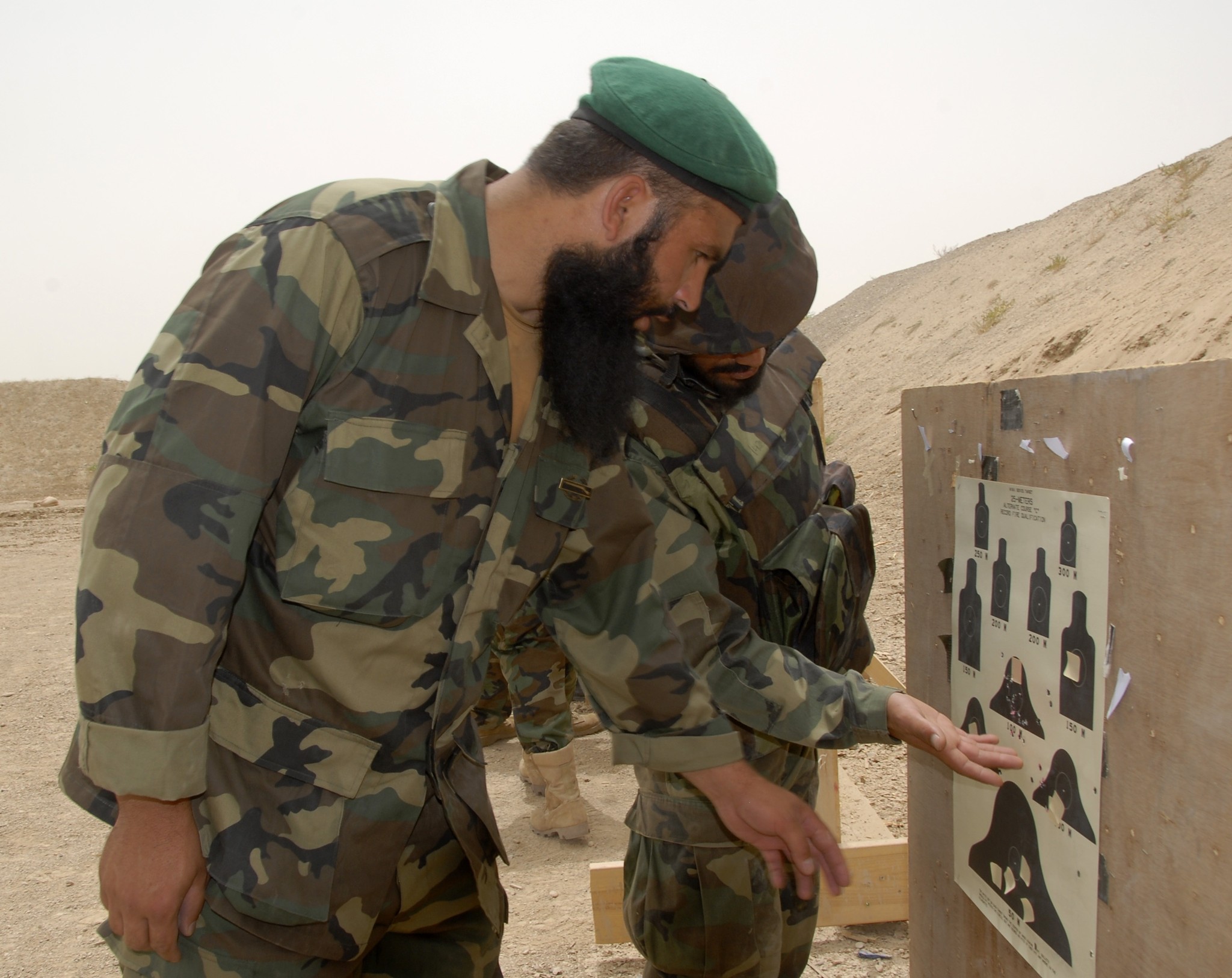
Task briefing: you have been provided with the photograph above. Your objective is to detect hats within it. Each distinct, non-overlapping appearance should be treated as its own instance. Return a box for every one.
[568,56,776,218]
[638,184,820,356]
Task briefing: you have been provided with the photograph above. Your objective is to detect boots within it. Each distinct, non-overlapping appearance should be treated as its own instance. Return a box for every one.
[518,749,546,797]
[527,737,591,841]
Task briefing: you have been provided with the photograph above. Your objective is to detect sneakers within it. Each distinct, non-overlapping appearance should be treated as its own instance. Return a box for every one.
[570,709,603,736]
[475,718,518,747]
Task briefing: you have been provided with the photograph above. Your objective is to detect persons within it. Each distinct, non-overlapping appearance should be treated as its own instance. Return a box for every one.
[472,598,605,841]
[59,55,780,978]
[539,189,1025,978]
